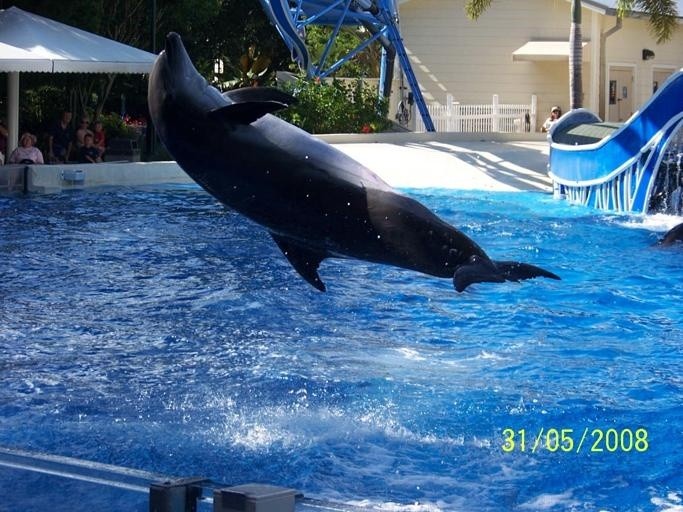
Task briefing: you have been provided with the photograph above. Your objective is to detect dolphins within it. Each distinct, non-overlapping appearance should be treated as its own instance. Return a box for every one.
[147,32,562,293]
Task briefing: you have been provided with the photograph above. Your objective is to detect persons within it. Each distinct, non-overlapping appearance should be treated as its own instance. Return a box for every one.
[540,105,561,133]
[0,109,104,166]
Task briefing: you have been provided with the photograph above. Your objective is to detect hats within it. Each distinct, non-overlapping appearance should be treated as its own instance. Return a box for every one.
[549,105,562,113]
[20,132,38,145]
